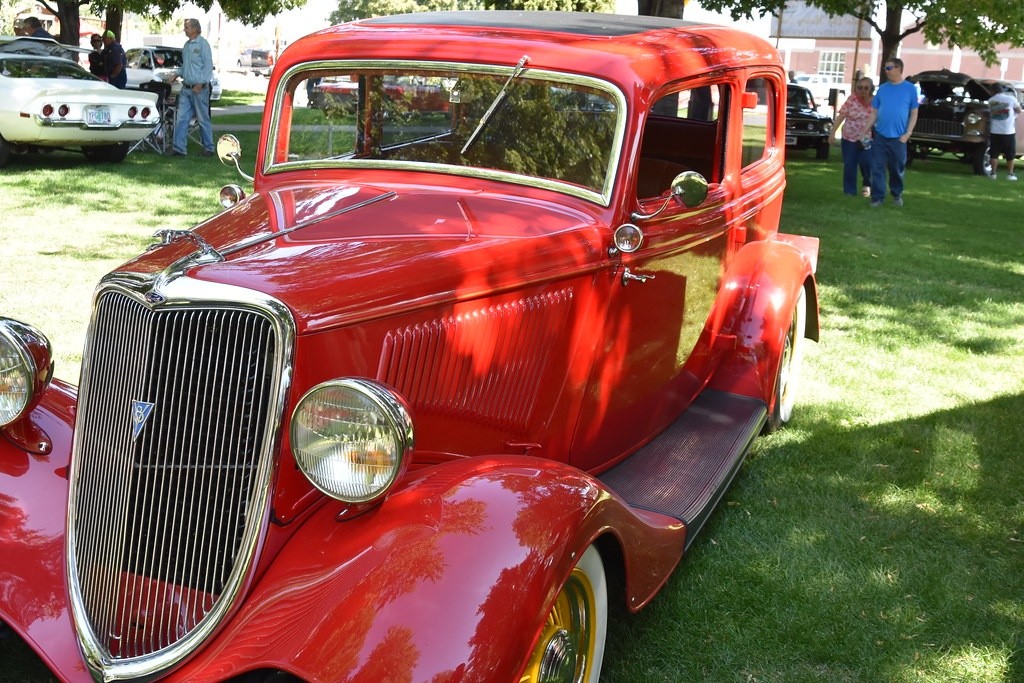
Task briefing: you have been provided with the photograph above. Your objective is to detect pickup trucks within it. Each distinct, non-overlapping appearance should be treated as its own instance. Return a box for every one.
[312,75,454,119]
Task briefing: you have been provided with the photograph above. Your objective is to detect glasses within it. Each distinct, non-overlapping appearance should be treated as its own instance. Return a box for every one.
[858,85,869,91]
[93,40,101,42]
[884,66,897,70]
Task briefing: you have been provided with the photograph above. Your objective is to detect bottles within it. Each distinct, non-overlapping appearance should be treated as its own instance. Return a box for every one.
[862,136,871,150]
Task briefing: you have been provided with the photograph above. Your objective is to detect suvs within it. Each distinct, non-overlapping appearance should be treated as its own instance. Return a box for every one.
[238,50,275,79]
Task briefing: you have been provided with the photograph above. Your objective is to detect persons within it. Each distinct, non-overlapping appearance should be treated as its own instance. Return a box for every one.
[171,18,216,157]
[306,77,324,107]
[88,30,127,89]
[12,17,54,38]
[988,83,1022,181]
[827,56,920,207]
[789,71,798,84]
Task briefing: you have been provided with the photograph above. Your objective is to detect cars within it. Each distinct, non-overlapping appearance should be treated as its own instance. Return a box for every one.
[0,9,820,683]
[786,84,834,160]
[122,49,223,97]
[0,35,160,165]
[900,71,1024,174]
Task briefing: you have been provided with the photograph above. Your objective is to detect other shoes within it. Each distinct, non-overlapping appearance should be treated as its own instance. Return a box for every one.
[871,199,880,206]
[169,150,179,156]
[990,173,996,179]
[200,150,212,157]
[862,186,870,197]
[1007,175,1017,180]
[894,195,902,205]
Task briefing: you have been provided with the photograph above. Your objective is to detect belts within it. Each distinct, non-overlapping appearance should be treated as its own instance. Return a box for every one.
[184,82,205,89]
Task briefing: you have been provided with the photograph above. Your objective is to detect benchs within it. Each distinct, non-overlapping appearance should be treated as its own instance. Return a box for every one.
[451,104,718,184]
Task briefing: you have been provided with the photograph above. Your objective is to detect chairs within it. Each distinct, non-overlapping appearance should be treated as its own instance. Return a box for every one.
[130,82,213,154]
[548,152,690,200]
[385,141,531,177]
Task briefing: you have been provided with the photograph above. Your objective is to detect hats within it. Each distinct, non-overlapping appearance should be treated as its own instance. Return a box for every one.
[12,19,25,29]
[101,31,115,38]
